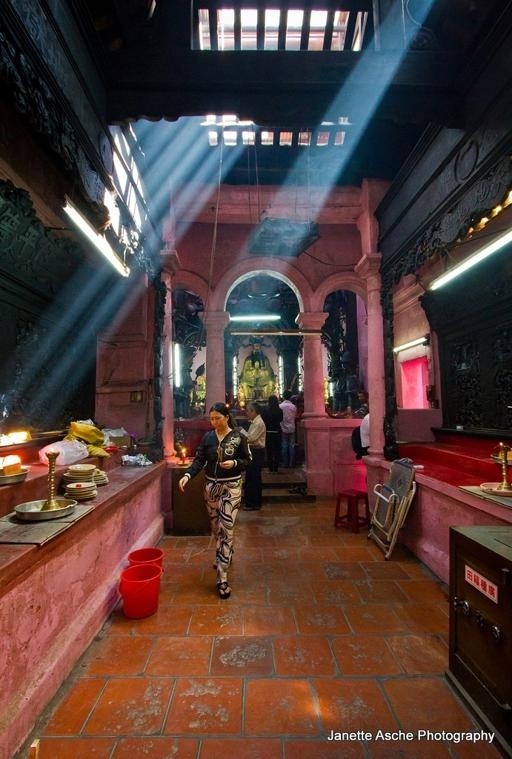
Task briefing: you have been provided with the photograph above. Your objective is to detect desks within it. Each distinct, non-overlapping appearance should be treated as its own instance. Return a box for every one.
[168,461,214,536]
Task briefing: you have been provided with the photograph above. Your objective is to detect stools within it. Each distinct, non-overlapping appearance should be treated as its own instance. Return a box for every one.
[333,488,371,533]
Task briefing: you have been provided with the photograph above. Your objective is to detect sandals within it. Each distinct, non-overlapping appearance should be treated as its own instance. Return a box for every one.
[213,555,232,569]
[217,582,231,599]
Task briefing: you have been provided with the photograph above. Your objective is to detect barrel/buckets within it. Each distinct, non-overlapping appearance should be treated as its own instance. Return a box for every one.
[118,563,165,620]
[128,547,164,570]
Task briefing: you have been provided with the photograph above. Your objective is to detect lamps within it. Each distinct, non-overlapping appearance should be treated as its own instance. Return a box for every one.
[391,333,430,355]
[171,341,182,390]
[429,225,512,291]
[60,191,131,279]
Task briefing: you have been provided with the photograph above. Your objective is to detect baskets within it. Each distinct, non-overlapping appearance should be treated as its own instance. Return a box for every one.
[109,435,130,446]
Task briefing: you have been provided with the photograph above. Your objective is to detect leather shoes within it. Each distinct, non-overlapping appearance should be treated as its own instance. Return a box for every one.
[243,506,261,511]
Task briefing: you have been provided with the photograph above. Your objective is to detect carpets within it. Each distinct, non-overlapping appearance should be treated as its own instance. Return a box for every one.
[398,432,512,487]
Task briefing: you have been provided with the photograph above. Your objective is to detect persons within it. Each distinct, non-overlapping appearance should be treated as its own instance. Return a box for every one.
[263,390,298,472]
[179,402,253,600]
[352,389,368,418]
[235,401,266,511]
[238,335,277,400]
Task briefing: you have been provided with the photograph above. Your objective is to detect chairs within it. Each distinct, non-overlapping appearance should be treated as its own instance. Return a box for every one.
[367,459,417,559]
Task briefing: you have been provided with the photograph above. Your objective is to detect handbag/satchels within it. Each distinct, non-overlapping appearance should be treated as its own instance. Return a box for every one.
[248,448,269,468]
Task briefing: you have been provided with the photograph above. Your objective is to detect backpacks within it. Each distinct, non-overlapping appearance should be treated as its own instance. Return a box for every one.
[352,426,362,460]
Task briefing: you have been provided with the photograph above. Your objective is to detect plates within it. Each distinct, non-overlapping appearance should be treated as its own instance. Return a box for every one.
[62,462,108,501]
[0,470,28,484]
[15,499,76,520]
[481,482,512,496]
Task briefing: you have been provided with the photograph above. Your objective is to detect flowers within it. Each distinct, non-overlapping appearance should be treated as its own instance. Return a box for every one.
[125,430,139,454]
